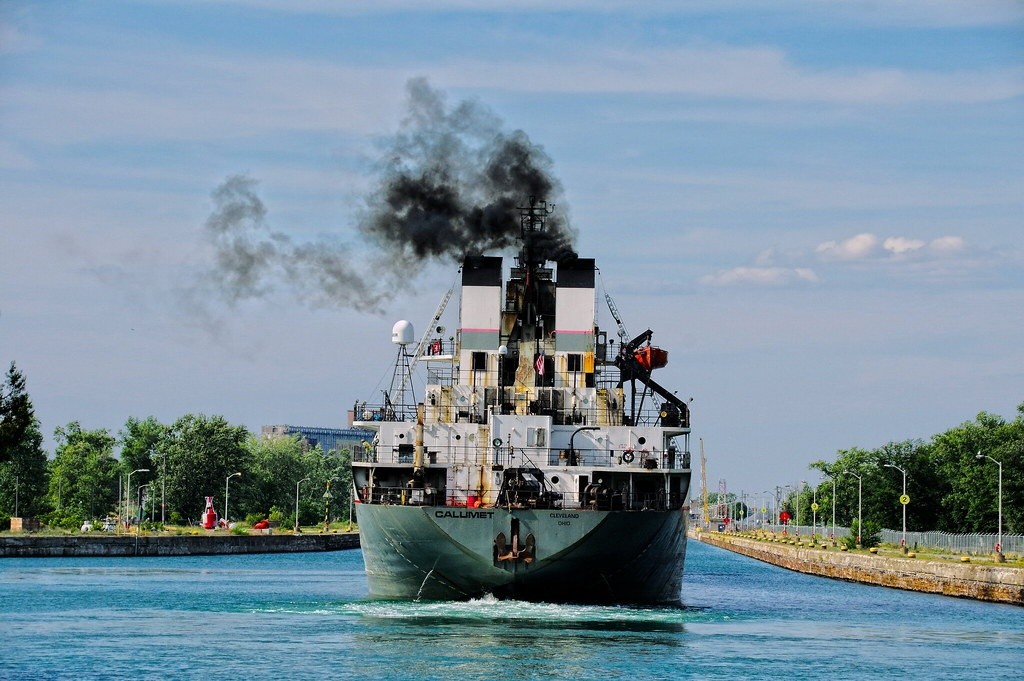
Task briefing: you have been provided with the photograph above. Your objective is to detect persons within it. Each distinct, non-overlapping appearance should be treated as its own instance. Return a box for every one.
[361,438,373,454]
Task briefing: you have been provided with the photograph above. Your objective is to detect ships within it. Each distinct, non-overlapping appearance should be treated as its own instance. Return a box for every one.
[348,203,692,608]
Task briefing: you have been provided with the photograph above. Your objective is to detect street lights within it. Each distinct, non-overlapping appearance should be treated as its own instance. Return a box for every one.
[125,469,150,533]
[762,490,777,538]
[754,492,765,533]
[293,477,311,532]
[784,485,800,543]
[819,476,837,547]
[225,472,242,530]
[975,455,1005,563]
[884,464,908,554]
[800,480,817,544]
[843,471,863,550]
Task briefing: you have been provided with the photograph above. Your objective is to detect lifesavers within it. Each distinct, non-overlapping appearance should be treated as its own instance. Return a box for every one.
[622,451,634,463]
